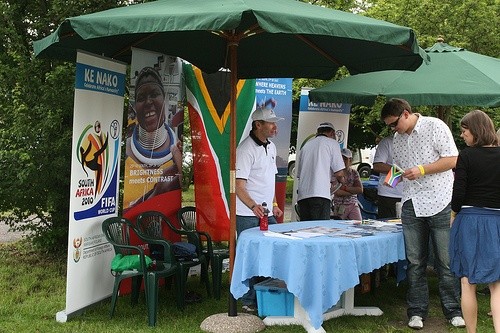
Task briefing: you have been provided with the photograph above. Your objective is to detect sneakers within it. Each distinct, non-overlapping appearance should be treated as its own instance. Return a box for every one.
[448,316,466,326]
[408,315,423,329]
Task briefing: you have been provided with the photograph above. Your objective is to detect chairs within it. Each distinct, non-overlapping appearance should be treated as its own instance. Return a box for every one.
[135,206,230,301]
[103,217,183,327]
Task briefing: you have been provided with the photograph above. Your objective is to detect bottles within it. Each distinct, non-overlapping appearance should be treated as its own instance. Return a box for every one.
[260,201,269,231]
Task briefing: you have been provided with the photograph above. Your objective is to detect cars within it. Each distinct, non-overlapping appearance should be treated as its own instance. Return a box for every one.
[288,144,378,180]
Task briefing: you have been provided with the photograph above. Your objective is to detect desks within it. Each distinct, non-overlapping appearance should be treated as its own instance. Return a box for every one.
[230,219,407,333]
[357,181,379,219]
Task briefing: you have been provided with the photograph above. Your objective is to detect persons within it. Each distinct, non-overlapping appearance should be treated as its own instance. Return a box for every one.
[476,286,492,317]
[331,149,363,220]
[236,109,283,312]
[122,67,182,209]
[449,110,500,332]
[373,127,403,219]
[297,122,346,221]
[381,98,466,329]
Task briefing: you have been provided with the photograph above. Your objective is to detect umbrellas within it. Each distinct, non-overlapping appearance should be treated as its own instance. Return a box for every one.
[33,0,431,286]
[307,36,500,120]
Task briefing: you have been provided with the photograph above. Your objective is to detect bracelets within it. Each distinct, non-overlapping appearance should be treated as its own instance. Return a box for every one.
[417,165,425,176]
[450,210,456,217]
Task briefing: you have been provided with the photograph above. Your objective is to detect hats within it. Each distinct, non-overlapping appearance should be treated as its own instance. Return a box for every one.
[341,148,352,158]
[251,107,285,123]
[318,121,335,131]
[135,68,164,92]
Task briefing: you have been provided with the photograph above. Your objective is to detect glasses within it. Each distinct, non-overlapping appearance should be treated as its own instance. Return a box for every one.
[389,113,401,127]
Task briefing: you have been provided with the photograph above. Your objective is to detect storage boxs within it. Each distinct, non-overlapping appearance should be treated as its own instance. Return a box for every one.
[254,278,295,316]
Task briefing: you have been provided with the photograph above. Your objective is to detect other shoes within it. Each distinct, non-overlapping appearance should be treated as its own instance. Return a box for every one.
[243,304,258,310]
[477,286,490,296]
